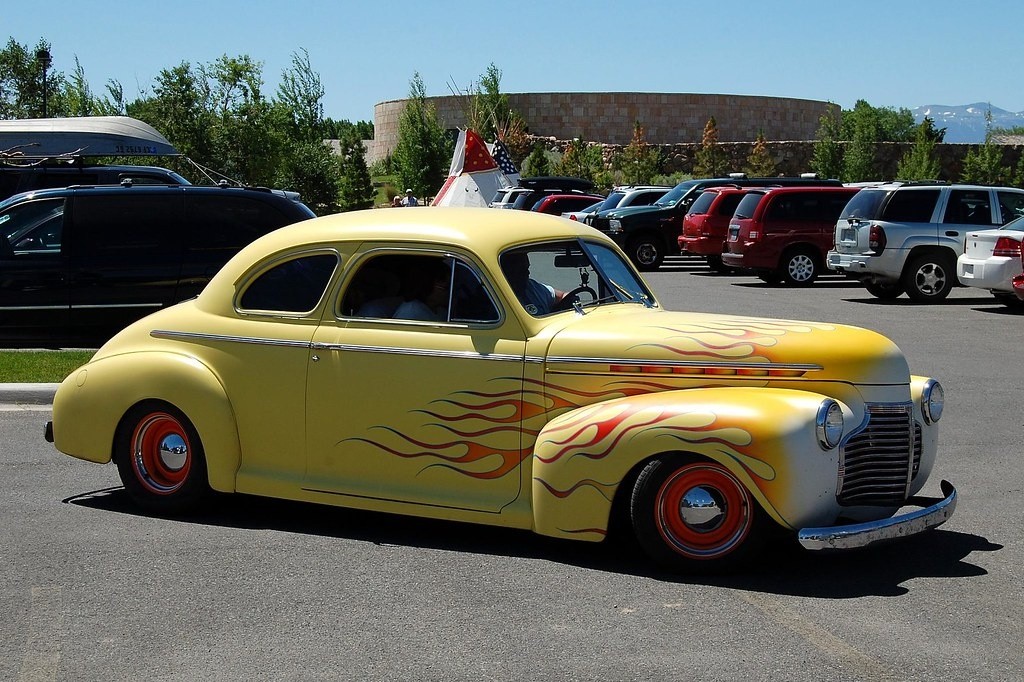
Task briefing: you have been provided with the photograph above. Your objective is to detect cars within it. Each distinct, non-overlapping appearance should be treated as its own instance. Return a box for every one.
[528,194,605,225]
[956,216,1023,309]
[44,205,961,575]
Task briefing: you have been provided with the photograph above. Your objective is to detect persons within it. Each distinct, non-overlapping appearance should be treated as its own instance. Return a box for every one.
[402,189,418,207]
[392,196,403,207]
[392,263,472,321]
[504,253,567,315]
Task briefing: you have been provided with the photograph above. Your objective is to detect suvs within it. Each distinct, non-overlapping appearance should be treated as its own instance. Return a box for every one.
[592,177,845,272]
[486,176,606,211]
[0,163,338,348]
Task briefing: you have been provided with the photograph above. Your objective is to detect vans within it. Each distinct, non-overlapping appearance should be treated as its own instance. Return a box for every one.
[721,180,1024,303]
